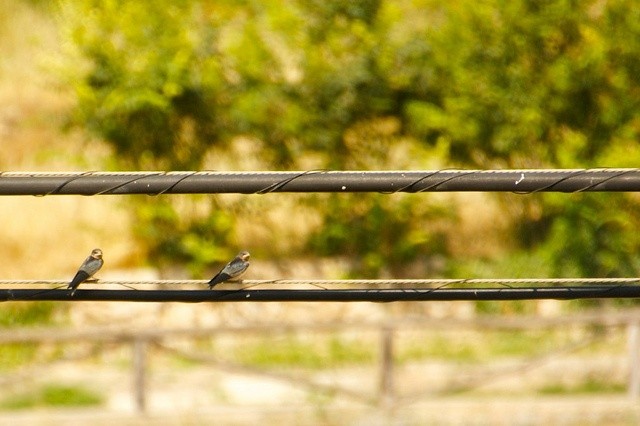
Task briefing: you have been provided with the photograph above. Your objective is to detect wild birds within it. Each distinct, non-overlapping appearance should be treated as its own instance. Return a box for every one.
[67,249,104,296]
[206,250,250,292]
